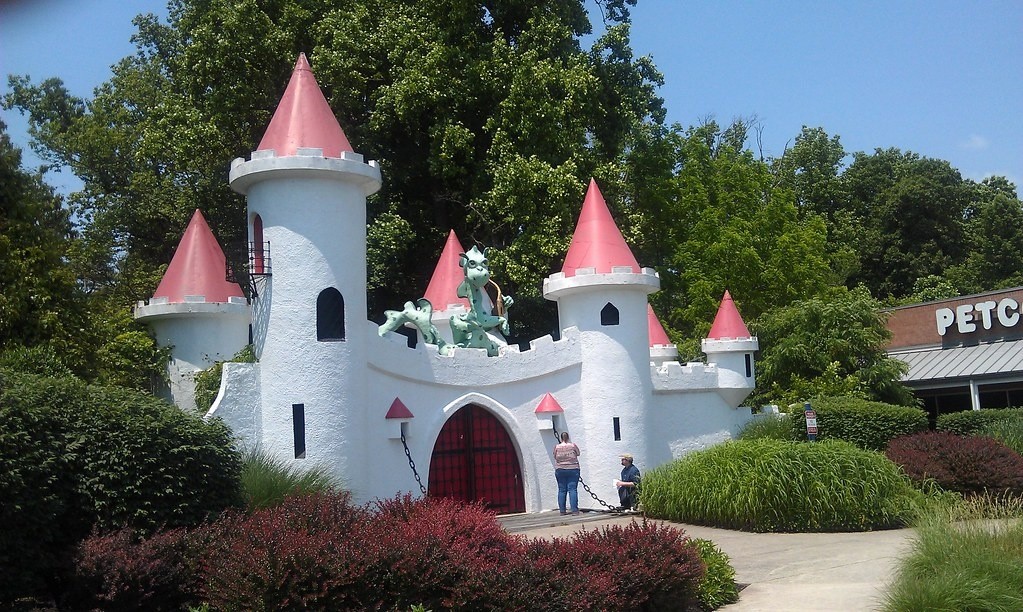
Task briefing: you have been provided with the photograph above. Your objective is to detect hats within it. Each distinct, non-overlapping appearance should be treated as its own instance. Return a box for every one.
[619,454,633,462]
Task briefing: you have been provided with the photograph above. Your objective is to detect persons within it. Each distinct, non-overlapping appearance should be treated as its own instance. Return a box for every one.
[617,453,643,508]
[553,432,581,516]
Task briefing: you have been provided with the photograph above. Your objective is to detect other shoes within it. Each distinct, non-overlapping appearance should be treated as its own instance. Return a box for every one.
[572,511,583,515]
[560,512,568,515]
